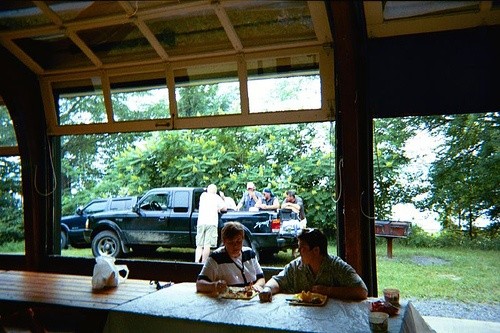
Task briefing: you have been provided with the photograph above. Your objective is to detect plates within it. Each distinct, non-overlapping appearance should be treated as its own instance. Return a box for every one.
[288,294,328,306]
[222,291,259,299]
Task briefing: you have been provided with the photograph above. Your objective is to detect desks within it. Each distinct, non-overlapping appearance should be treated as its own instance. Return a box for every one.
[106,281,413,333]
[0,270,175,332]
[402,299,499,332]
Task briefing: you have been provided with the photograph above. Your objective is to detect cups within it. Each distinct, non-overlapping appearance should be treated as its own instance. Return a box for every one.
[367,312,389,333]
[383,289,399,305]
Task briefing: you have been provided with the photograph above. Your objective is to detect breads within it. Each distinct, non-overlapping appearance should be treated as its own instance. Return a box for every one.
[301,290,311,303]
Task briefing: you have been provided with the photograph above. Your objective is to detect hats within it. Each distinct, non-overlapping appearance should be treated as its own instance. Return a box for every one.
[264,189,271,192]
[247,181,254,190]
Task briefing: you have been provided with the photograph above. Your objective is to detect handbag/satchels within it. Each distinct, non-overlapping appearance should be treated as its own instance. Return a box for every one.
[93,255,130,290]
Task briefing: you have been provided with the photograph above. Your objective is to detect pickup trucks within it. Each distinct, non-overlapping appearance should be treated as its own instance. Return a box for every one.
[84,186,302,265]
[61,196,141,250]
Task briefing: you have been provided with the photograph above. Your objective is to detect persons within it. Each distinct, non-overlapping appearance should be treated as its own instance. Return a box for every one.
[195,220,265,296]
[215,190,236,211]
[259,227,370,303]
[281,190,308,231]
[234,181,265,213]
[253,188,280,214]
[192,182,228,266]
